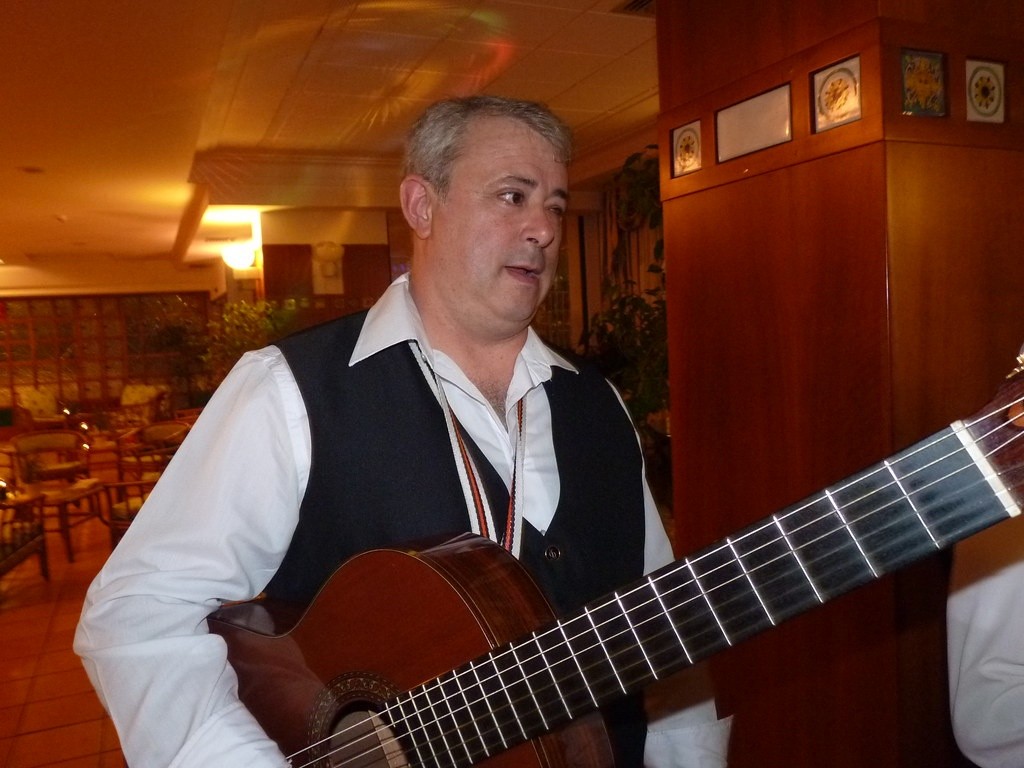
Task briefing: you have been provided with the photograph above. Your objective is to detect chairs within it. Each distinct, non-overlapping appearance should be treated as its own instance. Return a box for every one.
[0,384,204,581]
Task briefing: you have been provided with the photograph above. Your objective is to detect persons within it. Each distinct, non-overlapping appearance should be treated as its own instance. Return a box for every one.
[945,515,1024,768]
[72,94,737,767]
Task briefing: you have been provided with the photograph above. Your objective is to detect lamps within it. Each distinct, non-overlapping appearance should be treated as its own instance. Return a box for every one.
[314,242,345,276]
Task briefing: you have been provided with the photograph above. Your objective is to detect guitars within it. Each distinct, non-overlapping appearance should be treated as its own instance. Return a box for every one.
[206,343,1024,768]
